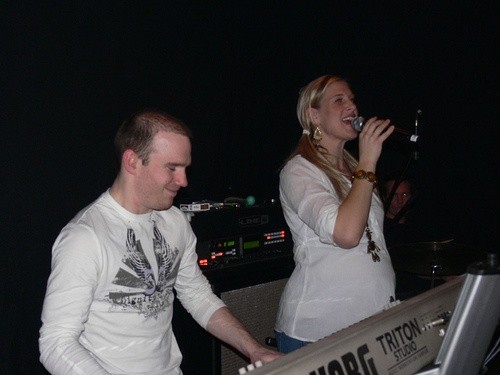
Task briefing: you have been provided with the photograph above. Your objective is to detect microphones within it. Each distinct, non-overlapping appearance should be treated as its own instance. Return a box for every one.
[351,117,423,144]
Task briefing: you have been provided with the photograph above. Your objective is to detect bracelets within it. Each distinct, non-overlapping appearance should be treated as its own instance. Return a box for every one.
[351,169,377,189]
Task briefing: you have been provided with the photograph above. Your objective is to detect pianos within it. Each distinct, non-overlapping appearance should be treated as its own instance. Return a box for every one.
[237,268,499,375]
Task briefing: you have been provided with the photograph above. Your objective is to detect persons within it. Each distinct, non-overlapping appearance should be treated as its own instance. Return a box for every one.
[274,76,396,353]
[38,111,286,375]
[382,175,460,300]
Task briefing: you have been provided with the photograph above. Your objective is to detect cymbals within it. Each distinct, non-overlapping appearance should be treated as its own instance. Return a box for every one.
[407,236,458,255]
